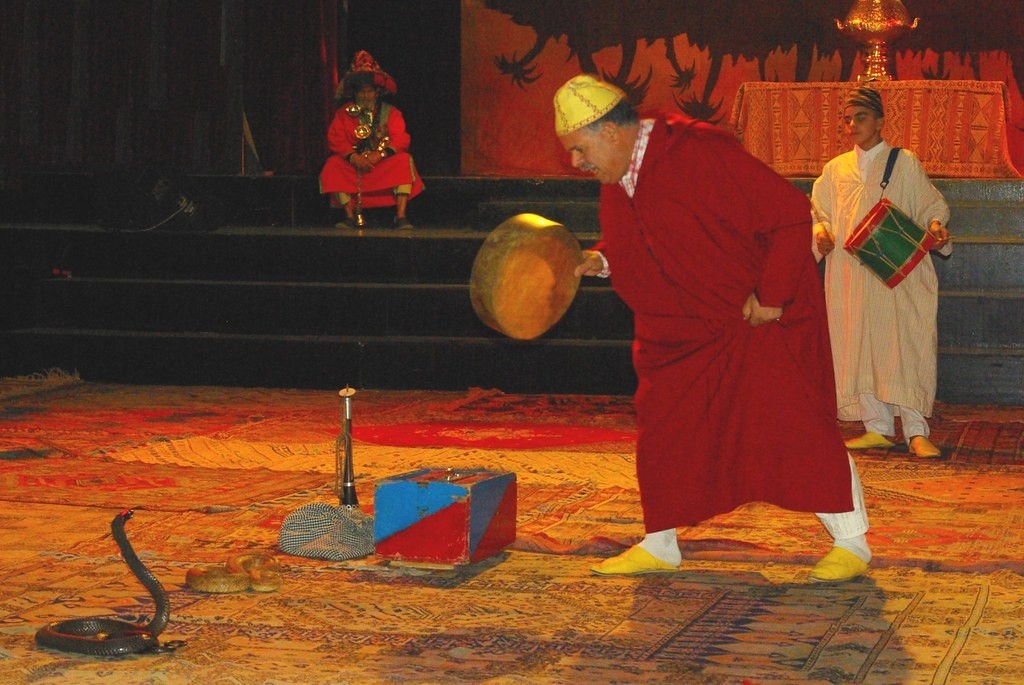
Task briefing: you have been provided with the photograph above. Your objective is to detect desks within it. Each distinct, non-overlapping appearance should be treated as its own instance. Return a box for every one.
[730,80,1021,178]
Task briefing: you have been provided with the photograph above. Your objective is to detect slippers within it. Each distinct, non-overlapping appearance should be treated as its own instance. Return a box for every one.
[808,547,871,583]
[590,544,679,575]
[845,433,896,449]
[907,437,942,459]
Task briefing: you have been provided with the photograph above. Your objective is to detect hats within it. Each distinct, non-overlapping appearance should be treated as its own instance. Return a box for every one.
[554,74,629,138]
[844,86,882,110]
[335,49,398,106]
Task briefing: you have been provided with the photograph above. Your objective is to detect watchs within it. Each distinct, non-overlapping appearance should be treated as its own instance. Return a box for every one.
[380,151,385,158]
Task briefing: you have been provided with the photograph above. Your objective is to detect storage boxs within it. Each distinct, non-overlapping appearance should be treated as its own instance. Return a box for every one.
[375,467,518,565]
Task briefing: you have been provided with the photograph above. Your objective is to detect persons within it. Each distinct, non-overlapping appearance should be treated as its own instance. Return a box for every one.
[317,50,425,228]
[553,76,876,583]
[810,87,953,457]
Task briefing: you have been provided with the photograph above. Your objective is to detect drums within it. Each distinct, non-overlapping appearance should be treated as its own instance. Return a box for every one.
[842,196,936,290]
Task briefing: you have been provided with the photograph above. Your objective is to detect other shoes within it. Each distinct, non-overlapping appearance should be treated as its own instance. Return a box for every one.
[334,216,363,230]
[395,217,415,229]
